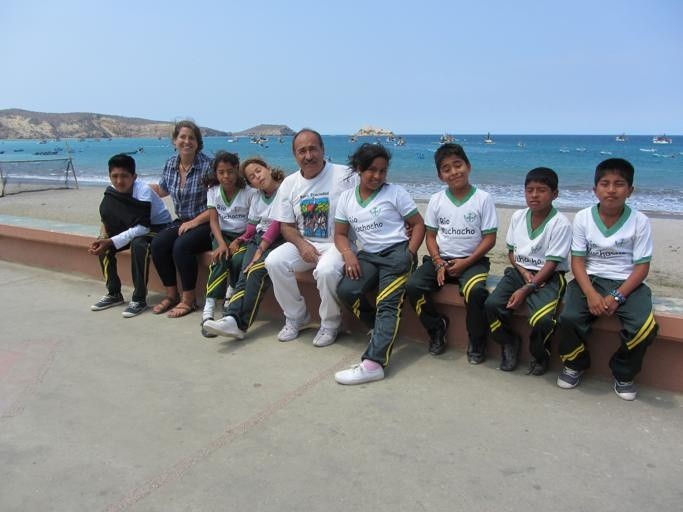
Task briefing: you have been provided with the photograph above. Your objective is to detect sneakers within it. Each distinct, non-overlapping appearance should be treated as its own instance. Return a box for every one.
[313,327,338,347]
[333,359,385,386]
[466,336,489,364]
[555,366,585,389]
[90,295,124,311]
[427,315,449,355]
[524,357,548,376]
[613,377,638,401]
[121,301,149,317]
[498,336,521,372]
[203,315,245,340]
[366,328,375,343]
[277,311,311,341]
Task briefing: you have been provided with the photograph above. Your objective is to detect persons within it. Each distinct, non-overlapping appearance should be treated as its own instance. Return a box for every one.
[335,143,424,385]
[484,167,574,377]
[265,129,414,347]
[145,122,220,317]
[201,153,257,338]
[406,143,498,365]
[87,155,174,318]
[203,159,287,340]
[558,159,660,400]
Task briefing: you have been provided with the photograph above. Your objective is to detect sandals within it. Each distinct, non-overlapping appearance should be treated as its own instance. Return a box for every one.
[152,292,181,314]
[166,300,196,318]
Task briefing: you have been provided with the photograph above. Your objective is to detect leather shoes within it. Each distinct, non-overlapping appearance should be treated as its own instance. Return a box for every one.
[199,316,218,339]
[222,297,231,311]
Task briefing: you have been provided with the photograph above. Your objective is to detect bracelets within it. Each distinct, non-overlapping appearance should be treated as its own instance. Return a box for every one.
[431,256,448,269]
[526,282,539,291]
[339,247,355,255]
[609,289,627,304]
